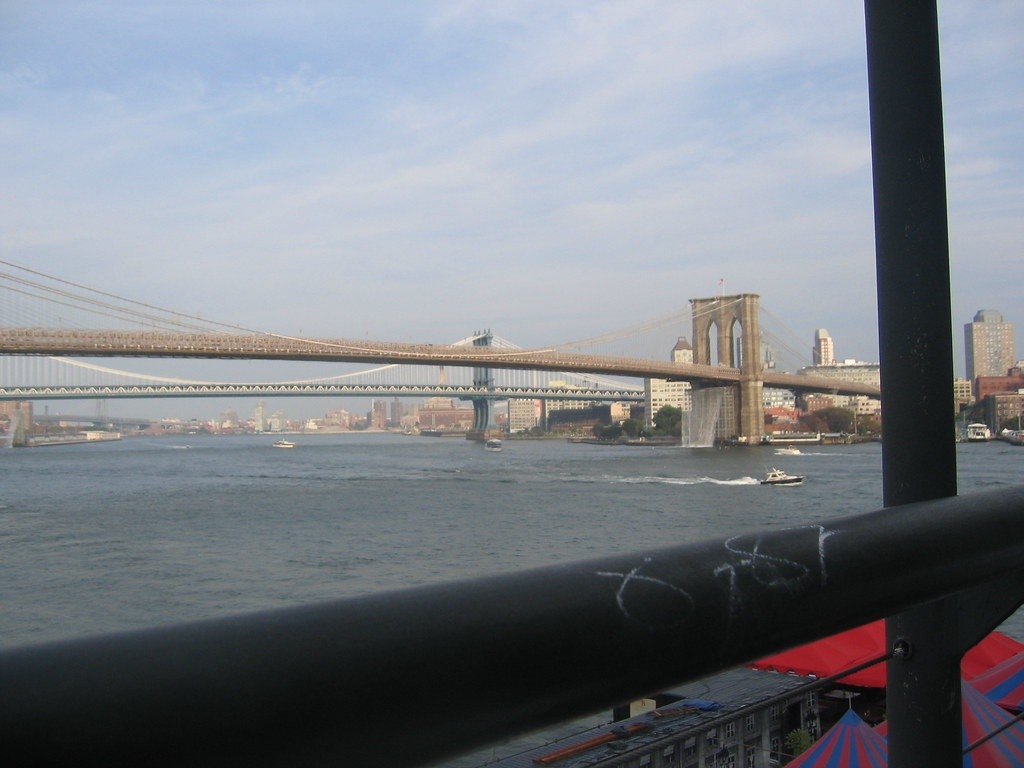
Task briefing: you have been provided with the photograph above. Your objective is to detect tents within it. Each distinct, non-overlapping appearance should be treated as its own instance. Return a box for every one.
[747,618,886,687]
[960,631,1024,712]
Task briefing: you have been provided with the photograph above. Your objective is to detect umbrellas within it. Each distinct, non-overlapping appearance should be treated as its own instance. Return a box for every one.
[961,676,1024,768]
[783,709,888,768]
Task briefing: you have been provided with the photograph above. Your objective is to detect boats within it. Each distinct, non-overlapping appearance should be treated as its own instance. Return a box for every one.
[484,438,503,452]
[967,423,992,441]
[760,467,805,485]
[272,438,295,448]
